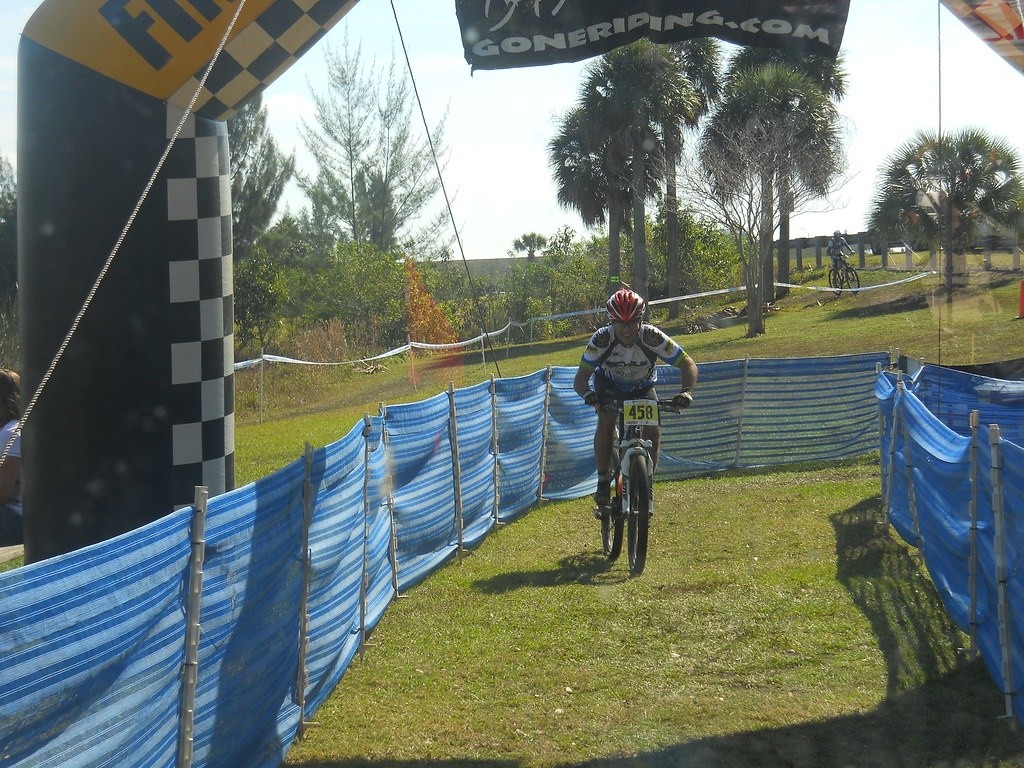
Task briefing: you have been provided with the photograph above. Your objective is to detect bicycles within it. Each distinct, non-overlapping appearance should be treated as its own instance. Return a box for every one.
[828,254,860,295]
[584,397,684,575]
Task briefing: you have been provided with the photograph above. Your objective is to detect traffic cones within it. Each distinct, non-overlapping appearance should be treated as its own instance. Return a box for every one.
[1014,279,1024,319]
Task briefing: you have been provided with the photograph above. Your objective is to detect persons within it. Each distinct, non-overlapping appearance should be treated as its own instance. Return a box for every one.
[826,230,855,288]
[572,288,698,516]
[0,368,25,547]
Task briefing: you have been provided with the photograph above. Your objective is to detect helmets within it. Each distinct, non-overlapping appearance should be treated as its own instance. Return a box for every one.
[606,289,646,322]
[834,230,841,238]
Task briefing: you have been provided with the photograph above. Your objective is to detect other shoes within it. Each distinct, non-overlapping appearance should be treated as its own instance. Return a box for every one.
[594,480,610,506]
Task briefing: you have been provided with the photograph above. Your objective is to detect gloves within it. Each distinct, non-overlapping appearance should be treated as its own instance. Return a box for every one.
[582,390,601,414]
[669,390,694,415]
